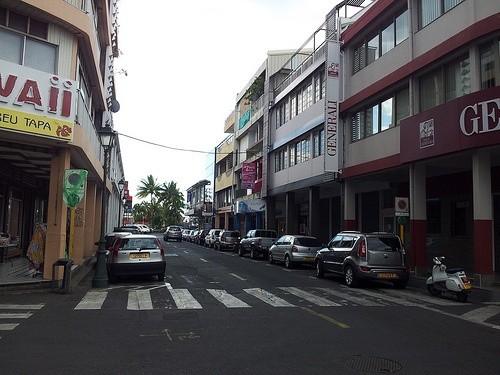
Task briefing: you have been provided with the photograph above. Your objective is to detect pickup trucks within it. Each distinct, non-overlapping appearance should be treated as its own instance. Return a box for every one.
[238,229,279,260]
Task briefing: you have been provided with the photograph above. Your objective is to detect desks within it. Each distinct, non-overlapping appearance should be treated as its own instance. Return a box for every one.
[0,244,16,267]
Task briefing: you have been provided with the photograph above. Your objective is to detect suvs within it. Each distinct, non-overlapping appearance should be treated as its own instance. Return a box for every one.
[314,230,411,289]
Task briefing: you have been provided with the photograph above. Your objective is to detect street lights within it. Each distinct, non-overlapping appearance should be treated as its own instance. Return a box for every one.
[117,179,126,228]
[92,123,118,289]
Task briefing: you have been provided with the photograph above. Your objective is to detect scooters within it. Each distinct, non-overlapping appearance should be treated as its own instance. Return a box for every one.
[425,256,473,302]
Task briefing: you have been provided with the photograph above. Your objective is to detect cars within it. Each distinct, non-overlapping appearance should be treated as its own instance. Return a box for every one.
[164,225,182,242]
[105,224,151,263]
[267,234,324,269]
[181,228,241,251]
[105,234,166,284]
